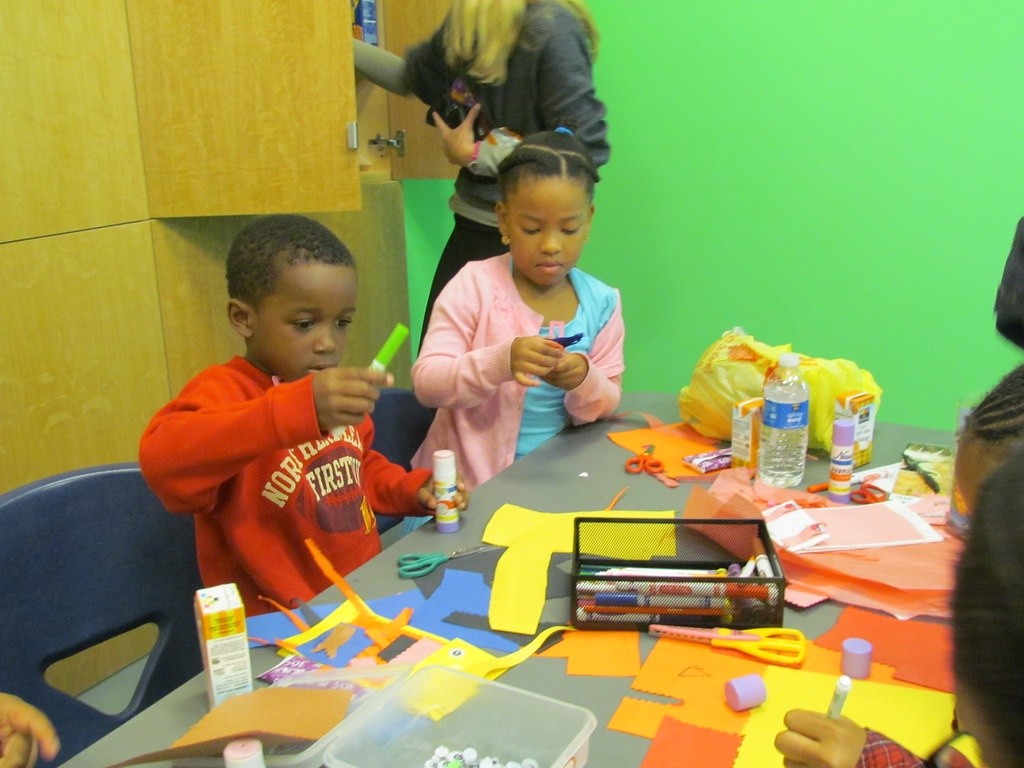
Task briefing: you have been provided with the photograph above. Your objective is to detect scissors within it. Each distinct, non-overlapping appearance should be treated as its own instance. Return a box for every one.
[850,483,888,505]
[624,443,664,473]
[396,543,493,579]
[648,625,808,666]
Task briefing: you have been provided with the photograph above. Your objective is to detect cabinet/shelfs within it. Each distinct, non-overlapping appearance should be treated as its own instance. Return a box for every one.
[0,0,466,700]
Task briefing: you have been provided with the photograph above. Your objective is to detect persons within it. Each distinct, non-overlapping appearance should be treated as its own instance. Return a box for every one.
[403,128,625,537]
[775,214,1024,767]
[353,0,610,358]
[140,214,470,616]
[0,692,61,768]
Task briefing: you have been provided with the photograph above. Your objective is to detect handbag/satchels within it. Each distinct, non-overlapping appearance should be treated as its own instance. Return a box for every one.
[681,329,881,470]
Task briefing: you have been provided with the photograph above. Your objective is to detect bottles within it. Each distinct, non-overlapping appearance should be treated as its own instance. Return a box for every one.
[758,351,810,487]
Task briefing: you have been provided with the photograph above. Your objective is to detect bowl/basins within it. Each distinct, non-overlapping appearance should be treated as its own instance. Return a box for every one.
[321,665,597,768]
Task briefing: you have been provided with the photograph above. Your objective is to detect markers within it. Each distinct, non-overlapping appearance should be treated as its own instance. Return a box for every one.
[849,476,851,477]
[327,323,410,440]
[806,470,889,492]
[574,537,778,624]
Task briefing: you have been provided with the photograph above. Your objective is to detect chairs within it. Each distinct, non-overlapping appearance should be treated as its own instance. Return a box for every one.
[365,386,438,536]
[0,461,202,768]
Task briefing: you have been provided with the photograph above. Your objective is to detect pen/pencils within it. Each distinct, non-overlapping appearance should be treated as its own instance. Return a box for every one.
[900,453,940,494]
[826,675,852,719]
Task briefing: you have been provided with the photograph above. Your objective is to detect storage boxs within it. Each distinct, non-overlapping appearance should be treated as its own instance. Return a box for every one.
[206,662,598,768]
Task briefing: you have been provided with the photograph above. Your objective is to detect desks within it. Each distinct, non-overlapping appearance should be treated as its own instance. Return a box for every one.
[61,390,978,768]
